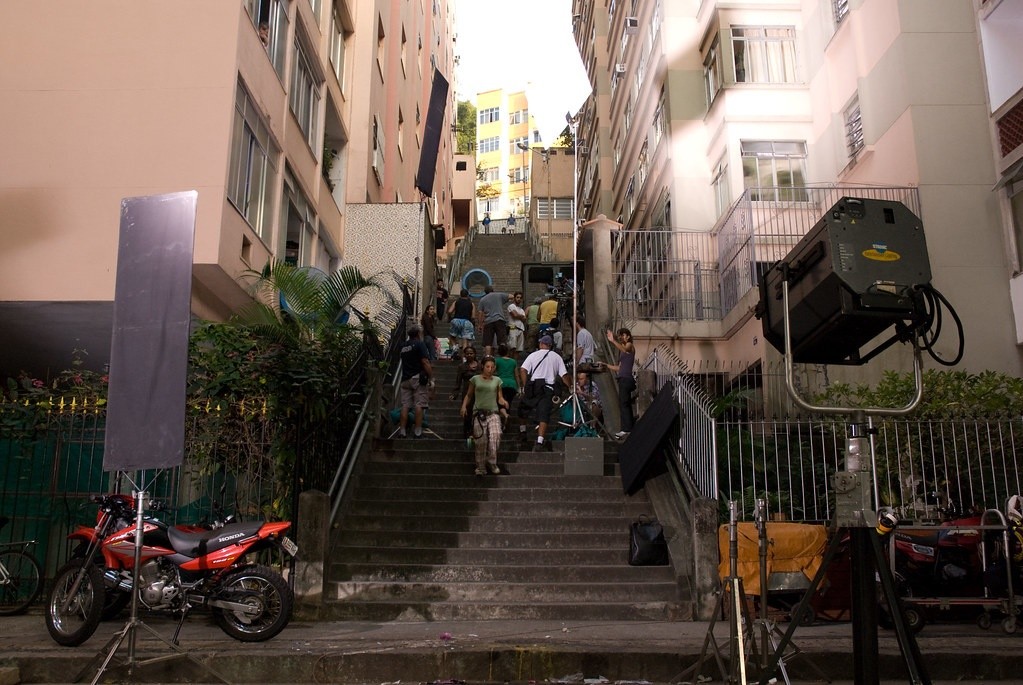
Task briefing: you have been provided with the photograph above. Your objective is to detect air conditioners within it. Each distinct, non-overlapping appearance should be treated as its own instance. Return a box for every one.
[581,147,588,157]
[453,33,456,41]
[583,199,591,208]
[615,64,626,77]
[455,55,460,62]
[626,17,638,30]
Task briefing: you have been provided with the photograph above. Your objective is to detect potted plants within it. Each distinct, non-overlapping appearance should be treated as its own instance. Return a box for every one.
[323,132,336,192]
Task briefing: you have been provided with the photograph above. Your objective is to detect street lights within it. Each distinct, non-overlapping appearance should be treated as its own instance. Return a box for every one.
[517,142,553,261]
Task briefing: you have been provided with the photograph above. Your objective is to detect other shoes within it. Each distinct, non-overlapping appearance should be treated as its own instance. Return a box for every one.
[536,441,545,449]
[399,434,406,439]
[488,462,500,473]
[502,415,512,432]
[615,431,632,439]
[476,471,484,476]
[517,431,527,440]
[451,350,458,358]
[413,433,422,439]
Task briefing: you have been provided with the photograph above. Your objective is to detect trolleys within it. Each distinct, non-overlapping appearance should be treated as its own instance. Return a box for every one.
[902,510,1016,633]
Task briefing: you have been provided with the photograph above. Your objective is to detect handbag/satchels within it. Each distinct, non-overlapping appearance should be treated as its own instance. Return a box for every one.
[629,514,668,566]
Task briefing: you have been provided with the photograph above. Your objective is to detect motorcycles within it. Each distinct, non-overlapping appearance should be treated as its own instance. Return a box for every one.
[44,494,299,647]
[864,496,1023,631]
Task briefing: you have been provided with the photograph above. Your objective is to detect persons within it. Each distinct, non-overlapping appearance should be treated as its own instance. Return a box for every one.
[570,370,603,431]
[572,316,598,369]
[258,23,269,46]
[538,293,558,338]
[448,289,475,354]
[448,346,483,441]
[459,357,509,476]
[524,297,543,356]
[506,291,526,361]
[422,305,436,361]
[482,213,490,235]
[507,213,516,237]
[436,278,449,324]
[520,335,572,452]
[597,328,637,434]
[398,326,433,439]
[478,286,515,357]
[492,345,522,431]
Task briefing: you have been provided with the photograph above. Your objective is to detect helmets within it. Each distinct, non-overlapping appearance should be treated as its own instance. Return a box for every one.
[538,336,552,345]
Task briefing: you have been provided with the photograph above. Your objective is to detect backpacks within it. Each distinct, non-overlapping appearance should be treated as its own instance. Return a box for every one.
[537,328,561,350]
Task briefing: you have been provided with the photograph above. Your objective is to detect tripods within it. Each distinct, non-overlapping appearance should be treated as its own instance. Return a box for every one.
[668,498,833,685]
[387,193,444,440]
[69,469,236,685]
[535,126,614,440]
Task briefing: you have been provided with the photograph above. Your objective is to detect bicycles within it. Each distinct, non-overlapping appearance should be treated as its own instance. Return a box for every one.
[0,514,45,617]
